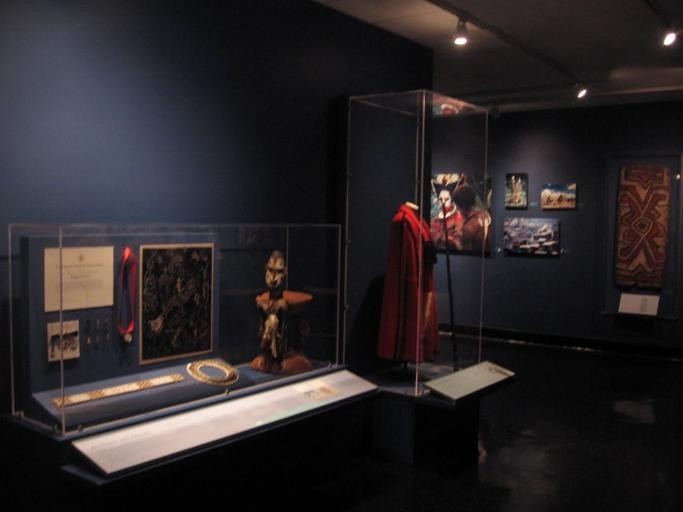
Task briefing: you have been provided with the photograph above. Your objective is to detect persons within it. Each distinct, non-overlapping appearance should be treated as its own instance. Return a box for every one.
[511,178,521,204]
[377,200,442,366]
[429,179,491,255]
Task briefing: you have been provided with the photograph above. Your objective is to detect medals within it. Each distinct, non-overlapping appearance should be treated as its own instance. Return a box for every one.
[124,333,132,343]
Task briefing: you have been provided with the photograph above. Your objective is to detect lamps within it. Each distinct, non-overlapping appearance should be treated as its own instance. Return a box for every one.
[571,79,589,100]
[453,10,470,46]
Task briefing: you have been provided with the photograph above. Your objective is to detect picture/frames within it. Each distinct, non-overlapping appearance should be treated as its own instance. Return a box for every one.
[138,242,215,365]
